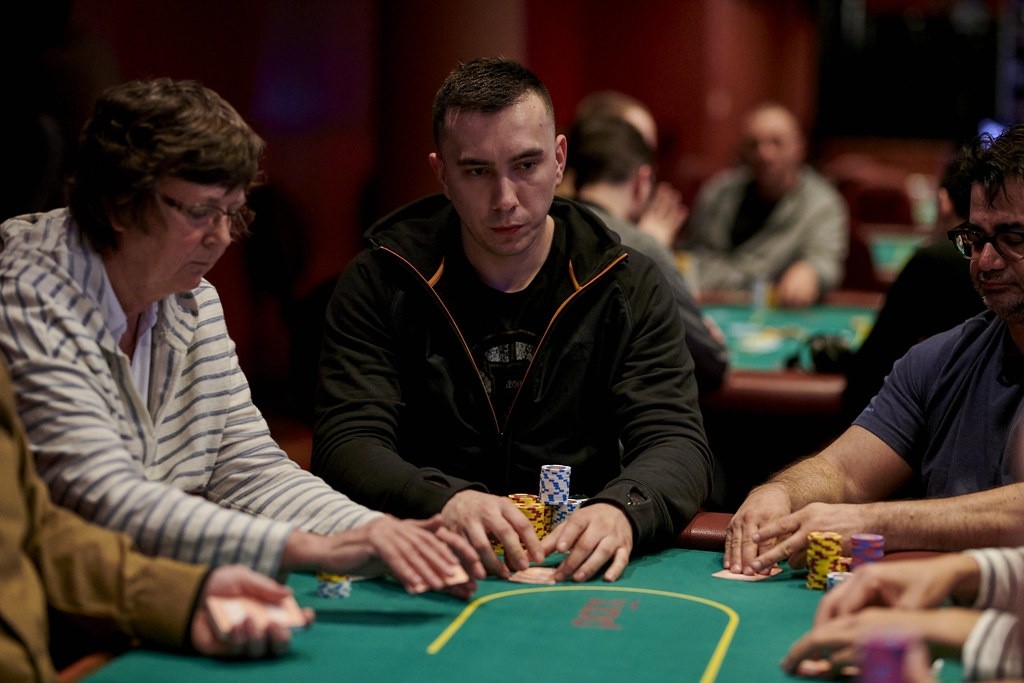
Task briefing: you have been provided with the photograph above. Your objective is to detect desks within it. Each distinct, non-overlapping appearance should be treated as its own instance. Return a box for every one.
[863,224,929,288]
[698,289,887,418]
[52,508,973,683]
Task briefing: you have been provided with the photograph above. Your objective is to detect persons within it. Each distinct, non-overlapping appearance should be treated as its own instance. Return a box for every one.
[0,354,315,683]
[554,91,730,382]
[237,179,311,315]
[689,103,850,304]
[783,545,1024,683]
[723,124,1024,575]
[0,77,485,599]
[837,156,985,421]
[311,57,715,583]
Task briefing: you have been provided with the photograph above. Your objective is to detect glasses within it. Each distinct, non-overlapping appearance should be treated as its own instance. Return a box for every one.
[947,221,1024,260]
[152,189,246,241]
